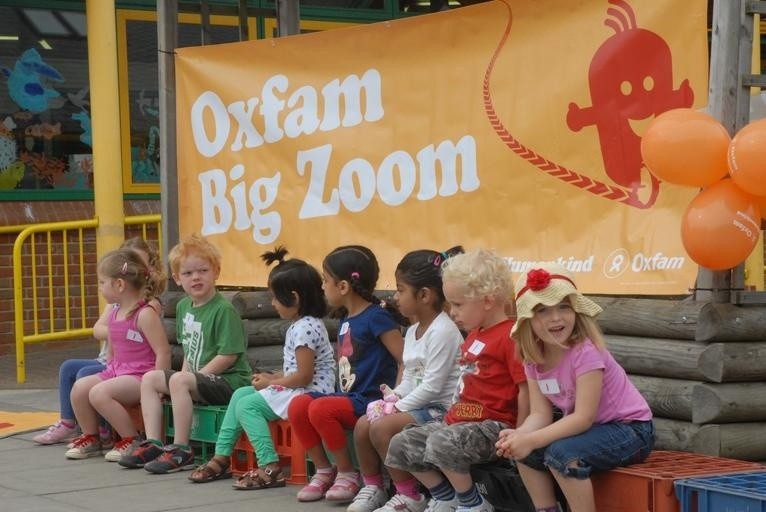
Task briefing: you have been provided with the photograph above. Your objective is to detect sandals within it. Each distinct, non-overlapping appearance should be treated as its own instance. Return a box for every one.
[232,465,286,491]
[186,457,234,483]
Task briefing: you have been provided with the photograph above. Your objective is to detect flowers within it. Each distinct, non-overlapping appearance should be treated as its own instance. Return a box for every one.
[526,268,551,291]
[271,384,285,392]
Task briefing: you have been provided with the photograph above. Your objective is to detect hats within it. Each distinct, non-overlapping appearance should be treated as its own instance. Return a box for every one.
[508,263,604,341]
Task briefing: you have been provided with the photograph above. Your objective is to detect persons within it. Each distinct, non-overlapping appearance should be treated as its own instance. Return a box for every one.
[33,235,166,450]
[288,245,404,503]
[188,243,337,490]
[345,245,466,512]
[119,233,252,475]
[65,248,171,463]
[383,248,531,512]
[495,265,655,512]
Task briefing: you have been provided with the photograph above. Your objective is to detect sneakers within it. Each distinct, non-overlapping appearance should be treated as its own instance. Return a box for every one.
[423,494,459,512]
[103,439,137,462]
[65,434,102,460]
[297,469,338,502]
[144,445,195,474]
[67,428,113,449]
[456,494,495,512]
[325,471,363,504]
[373,491,428,512]
[345,483,388,512]
[33,420,79,445]
[118,440,165,469]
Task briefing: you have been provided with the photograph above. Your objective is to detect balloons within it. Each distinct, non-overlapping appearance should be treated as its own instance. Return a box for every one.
[639,106,731,187]
[679,177,763,272]
[725,117,766,196]
